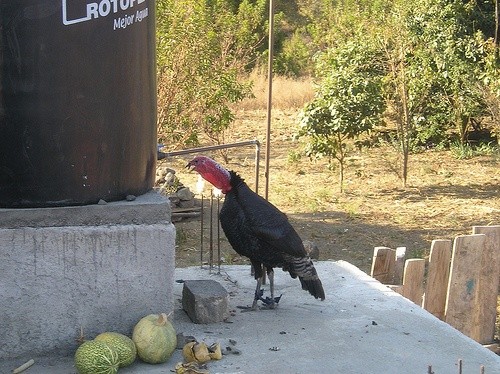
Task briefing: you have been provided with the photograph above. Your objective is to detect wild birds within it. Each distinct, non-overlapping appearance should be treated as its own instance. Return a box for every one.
[185,156,326,313]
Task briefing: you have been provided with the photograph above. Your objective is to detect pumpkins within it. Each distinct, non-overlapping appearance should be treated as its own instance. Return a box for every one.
[133,314,177,364]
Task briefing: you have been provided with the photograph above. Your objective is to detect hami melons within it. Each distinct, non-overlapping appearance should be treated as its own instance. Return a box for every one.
[75,332,136,374]
